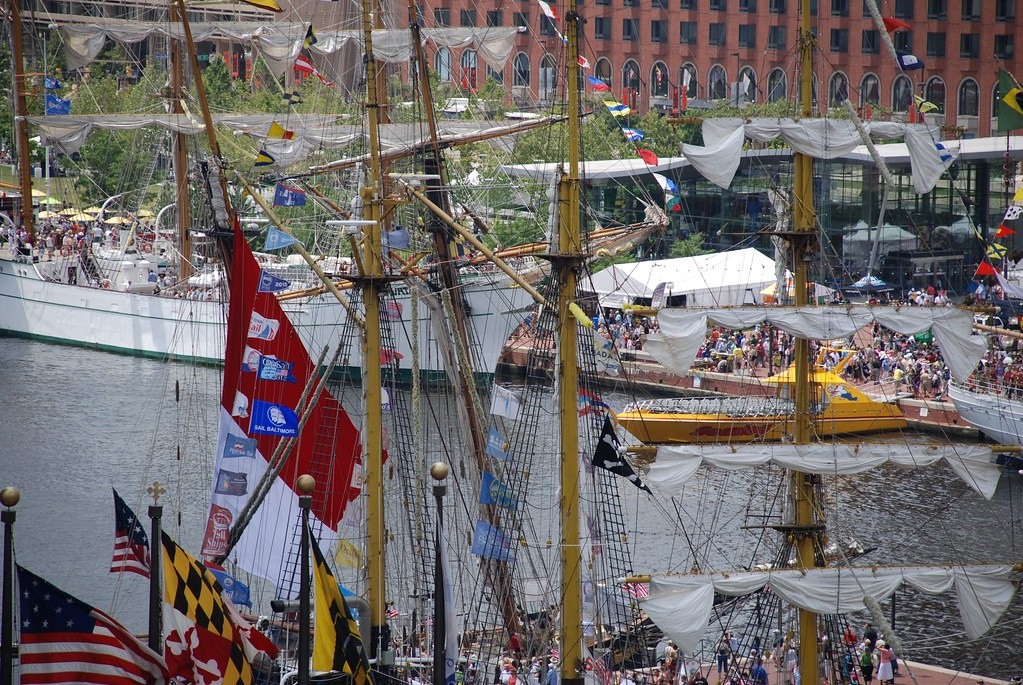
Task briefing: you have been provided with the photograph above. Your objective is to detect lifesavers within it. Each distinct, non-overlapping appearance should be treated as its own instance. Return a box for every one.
[61,247,70,256]
[144,243,152,253]
[102,280,111,288]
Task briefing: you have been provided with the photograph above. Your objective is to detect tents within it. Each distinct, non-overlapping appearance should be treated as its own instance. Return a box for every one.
[844,217,977,256]
[578,247,893,305]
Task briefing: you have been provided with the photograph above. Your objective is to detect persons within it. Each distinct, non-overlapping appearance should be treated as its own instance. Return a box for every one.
[0,147,215,301]
[509,284,1023,403]
[391,622,902,685]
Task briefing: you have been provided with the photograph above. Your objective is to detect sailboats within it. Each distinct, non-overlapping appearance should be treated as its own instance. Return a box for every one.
[0,0,1023,685]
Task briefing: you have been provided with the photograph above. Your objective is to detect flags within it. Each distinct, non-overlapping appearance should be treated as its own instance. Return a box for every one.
[294,53,334,87]
[0,181,405,685]
[882,17,1023,300]
[621,581,649,599]
[240,122,310,294]
[470,382,525,563]
[43,76,71,116]
[536,0,681,211]
